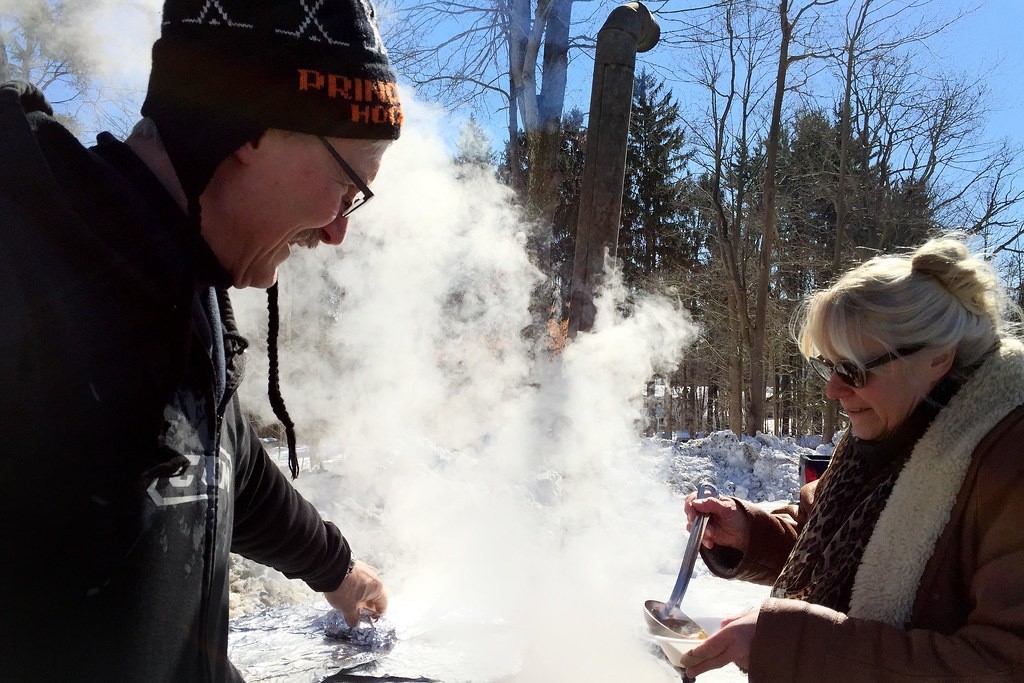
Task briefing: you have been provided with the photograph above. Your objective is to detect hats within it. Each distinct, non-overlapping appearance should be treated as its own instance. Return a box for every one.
[141,0,401,479]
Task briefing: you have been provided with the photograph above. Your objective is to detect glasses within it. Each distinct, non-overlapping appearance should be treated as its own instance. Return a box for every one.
[808,343,925,388]
[319,135,375,218]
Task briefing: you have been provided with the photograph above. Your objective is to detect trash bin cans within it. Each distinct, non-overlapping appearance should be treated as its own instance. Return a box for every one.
[799,454,832,485]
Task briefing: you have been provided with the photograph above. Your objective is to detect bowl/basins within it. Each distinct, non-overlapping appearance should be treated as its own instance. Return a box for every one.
[642,617,727,670]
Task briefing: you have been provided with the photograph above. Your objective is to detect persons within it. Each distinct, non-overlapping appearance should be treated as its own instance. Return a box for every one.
[680,231,1023,683]
[1,0,402,682]
